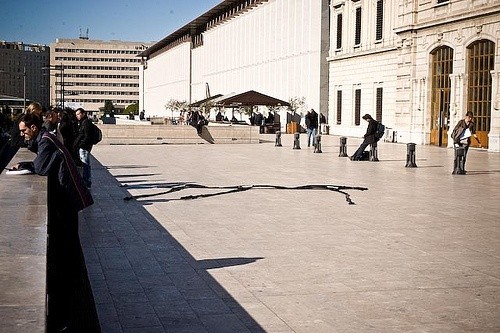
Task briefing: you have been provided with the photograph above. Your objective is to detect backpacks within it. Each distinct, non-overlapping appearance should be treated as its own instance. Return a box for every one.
[375,123,385,142]
[82,118,102,145]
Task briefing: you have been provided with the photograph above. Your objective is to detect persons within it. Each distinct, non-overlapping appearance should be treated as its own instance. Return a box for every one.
[179,107,209,136]
[347,114,379,161]
[139,109,145,120]
[305,109,318,147]
[215,112,224,121]
[450,111,477,173]
[249,109,275,134]
[319,113,326,124]
[0,101,99,287]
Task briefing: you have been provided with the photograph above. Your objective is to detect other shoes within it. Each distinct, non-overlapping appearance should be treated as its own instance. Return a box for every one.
[308,145,310,147]
[461,171,468,174]
[87,184,91,188]
[348,155,355,161]
[312,144,314,146]
[465,171,467,172]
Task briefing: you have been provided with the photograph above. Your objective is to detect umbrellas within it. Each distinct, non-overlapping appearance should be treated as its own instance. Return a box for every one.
[187,89,291,126]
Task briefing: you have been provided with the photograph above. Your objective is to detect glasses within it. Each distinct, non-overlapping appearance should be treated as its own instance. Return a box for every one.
[365,119,367,120]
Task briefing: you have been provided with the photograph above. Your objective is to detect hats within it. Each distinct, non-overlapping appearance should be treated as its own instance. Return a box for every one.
[362,114,372,119]
